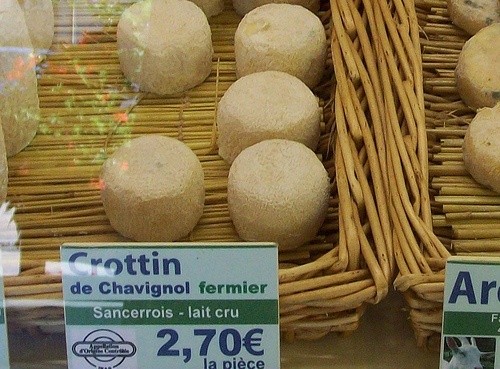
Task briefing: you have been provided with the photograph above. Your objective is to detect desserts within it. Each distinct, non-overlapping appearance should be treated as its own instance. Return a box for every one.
[234,4,326,90]
[0,0,54,205]
[218,71,320,165]
[463,102,500,195]
[187,0,226,18]
[449,0,500,37]
[455,22,500,113]
[228,139,330,251]
[117,0,212,95]
[98,136,204,242]
[233,0,320,18]
